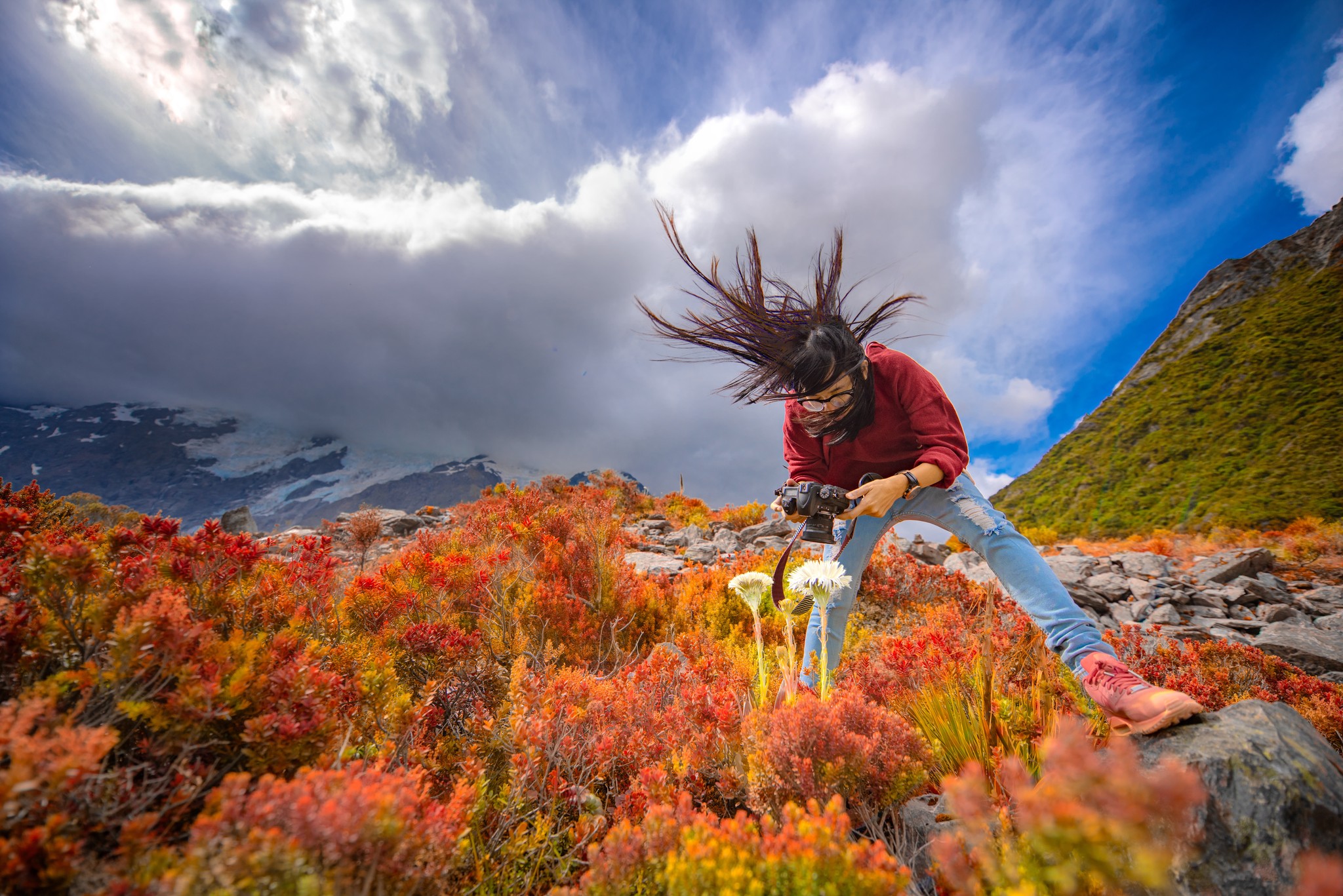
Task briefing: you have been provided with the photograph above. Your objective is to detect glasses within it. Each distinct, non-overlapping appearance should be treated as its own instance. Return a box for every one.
[796,368,859,412]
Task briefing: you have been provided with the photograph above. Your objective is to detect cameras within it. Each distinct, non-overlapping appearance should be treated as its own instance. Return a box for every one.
[781,481,851,544]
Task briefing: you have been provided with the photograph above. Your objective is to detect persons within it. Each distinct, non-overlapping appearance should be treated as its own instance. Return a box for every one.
[636,201,1205,736]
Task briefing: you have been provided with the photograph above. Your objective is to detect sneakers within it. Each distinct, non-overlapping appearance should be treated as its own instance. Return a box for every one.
[1080,652,1204,738]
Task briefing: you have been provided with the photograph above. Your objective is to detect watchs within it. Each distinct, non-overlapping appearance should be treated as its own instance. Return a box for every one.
[894,470,921,500]
